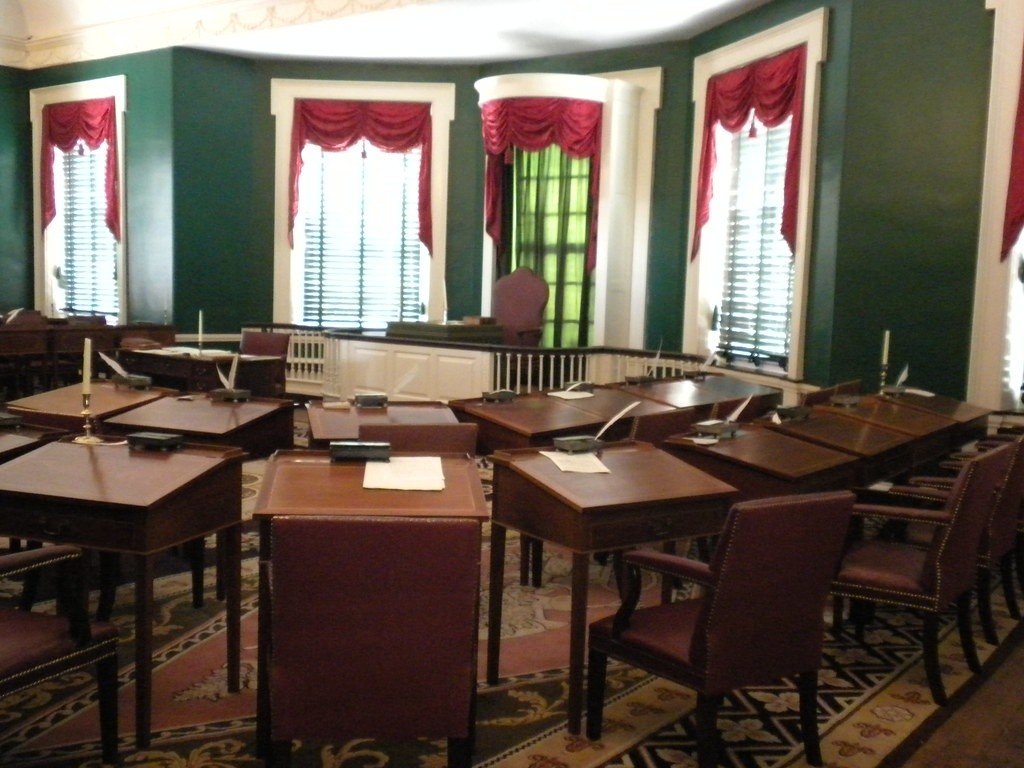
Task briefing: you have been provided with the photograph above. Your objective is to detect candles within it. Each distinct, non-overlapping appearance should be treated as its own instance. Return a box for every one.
[881,329,890,364]
[198,309,203,342]
[82,337,91,393]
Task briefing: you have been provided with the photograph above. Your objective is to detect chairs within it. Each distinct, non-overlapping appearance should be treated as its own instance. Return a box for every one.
[358,422,478,457]
[481,266,550,353]
[585,490,857,768]
[593,406,696,566]
[239,331,292,398]
[799,385,837,409]
[64,316,111,380]
[262,515,482,768]
[889,435,1024,647]
[3,307,53,397]
[708,395,761,424]
[830,441,1017,708]
[0,544,122,768]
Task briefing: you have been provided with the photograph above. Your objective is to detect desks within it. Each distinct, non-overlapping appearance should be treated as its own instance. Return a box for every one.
[0,324,176,400]
[0,422,69,582]
[117,345,286,398]
[251,449,491,760]
[606,371,784,425]
[663,425,862,635]
[304,399,460,451]
[485,439,738,737]
[755,412,915,485]
[813,396,958,473]
[448,391,603,587]
[542,383,678,442]
[103,390,296,601]
[0,432,249,751]
[5,379,178,434]
[879,393,993,444]
[385,320,508,390]
